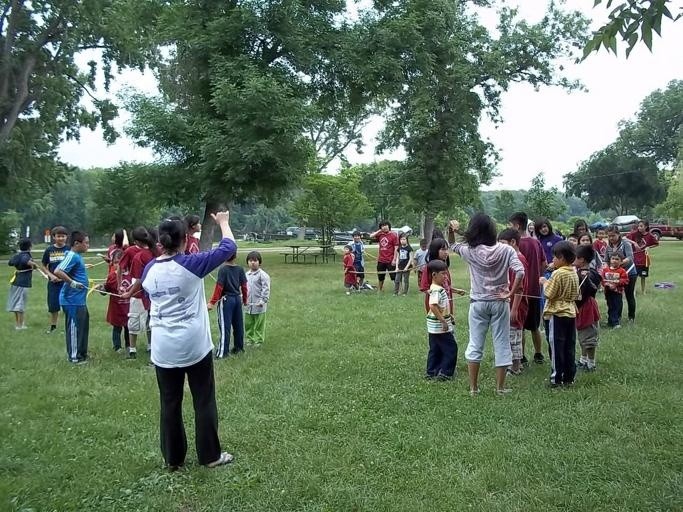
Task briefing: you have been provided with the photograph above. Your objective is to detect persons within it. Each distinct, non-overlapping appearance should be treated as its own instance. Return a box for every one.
[41,227,94,334]
[140,211,237,469]
[393,233,413,296]
[207,252,247,359]
[54,231,94,364]
[346,232,365,289]
[370,221,398,294]
[8,240,38,330]
[239,252,270,347]
[343,245,360,295]
[100,215,237,354]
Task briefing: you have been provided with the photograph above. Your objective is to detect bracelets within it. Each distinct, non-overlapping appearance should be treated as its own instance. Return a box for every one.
[69,281,77,289]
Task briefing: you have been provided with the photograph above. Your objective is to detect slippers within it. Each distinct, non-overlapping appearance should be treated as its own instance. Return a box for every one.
[201,451,236,467]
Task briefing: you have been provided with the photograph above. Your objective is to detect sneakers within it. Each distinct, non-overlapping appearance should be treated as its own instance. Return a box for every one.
[47,327,57,334]
[14,325,28,331]
[468,389,483,395]
[546,378,573,388]
[126,349,138,362]
[577,358,596,373]
[505,354,544,376]
[495,389,514,395]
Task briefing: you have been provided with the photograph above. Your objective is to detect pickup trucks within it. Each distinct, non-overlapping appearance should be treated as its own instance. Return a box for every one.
[648,217,683,240]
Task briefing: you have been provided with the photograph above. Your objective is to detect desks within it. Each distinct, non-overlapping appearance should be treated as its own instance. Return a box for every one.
[285,245,331,263]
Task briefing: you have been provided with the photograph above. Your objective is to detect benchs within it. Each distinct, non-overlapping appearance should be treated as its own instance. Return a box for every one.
[279,253,337,264]
